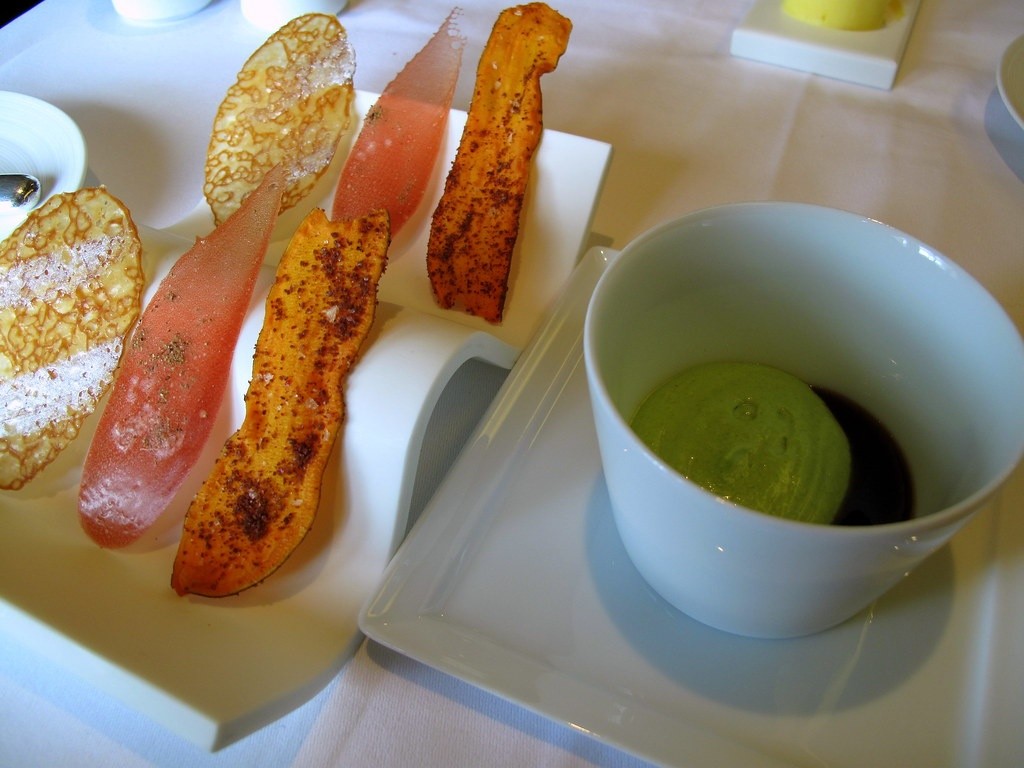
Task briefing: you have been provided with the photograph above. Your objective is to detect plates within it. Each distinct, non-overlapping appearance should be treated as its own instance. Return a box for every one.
[1,90,89,243]
[0,81,614,750]
[585,200,1024,641]
[357,245,1024,768]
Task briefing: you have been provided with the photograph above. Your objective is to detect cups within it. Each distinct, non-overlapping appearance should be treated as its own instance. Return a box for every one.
[110,0,212,22]
[239,0,351,31]
